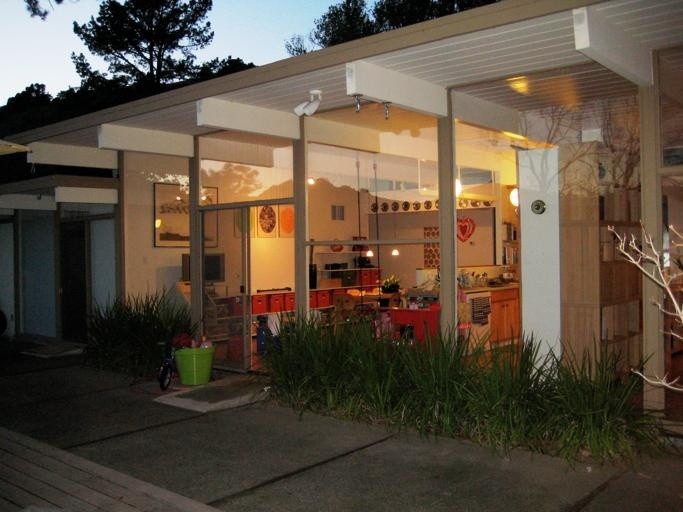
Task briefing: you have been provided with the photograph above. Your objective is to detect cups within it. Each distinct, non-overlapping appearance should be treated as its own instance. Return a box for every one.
[416,267,424,285]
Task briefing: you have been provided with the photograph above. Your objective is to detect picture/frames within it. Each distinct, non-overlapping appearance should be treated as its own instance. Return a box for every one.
[153,181,219,249]
[233,195,295,240]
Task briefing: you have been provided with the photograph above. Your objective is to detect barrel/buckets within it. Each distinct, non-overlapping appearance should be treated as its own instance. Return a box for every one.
[173,347,215,387]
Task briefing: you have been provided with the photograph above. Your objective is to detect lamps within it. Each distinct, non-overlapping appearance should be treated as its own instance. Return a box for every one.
[294,89,323,117]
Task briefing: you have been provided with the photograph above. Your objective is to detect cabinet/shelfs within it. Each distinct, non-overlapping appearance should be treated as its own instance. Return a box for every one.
[489,288,520,349]
[310,250,371,288]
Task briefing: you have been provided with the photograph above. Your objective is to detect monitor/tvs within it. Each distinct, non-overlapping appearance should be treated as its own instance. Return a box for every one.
[182,253,225,282]
